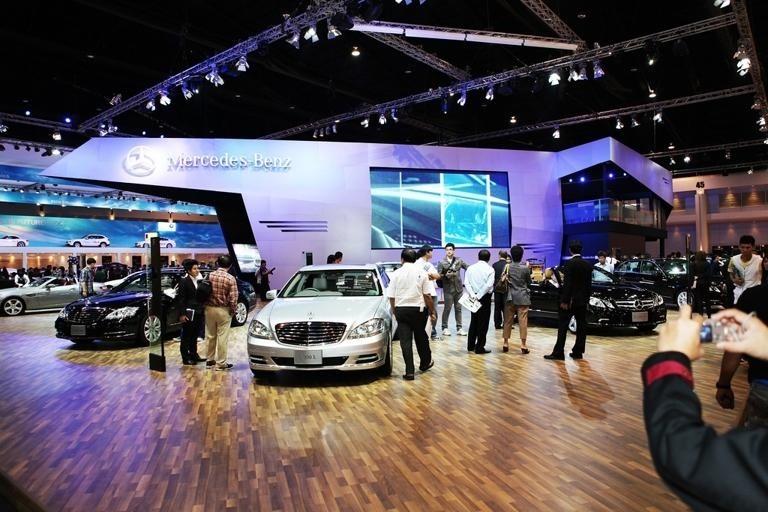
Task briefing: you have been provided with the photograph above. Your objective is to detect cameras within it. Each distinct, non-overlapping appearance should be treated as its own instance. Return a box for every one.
[700,320,724,342]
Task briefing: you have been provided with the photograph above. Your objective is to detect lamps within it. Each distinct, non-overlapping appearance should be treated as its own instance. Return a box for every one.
[114,94,122,103]
[351,46,360,57]
[578,68,588,81]
[159,94,171,105]
[524,40,577,51]
[378,114,387,125]
[457,96,467,107]
[39,206,45,217]
[52,130,62,141]
[235,56,249,71]
[109,209,115,220]
[348,24,404,35]
[97,128,107,136]
[467,34,524,47]
[109,97,116,106]
[145,99,156,111]
[485,88,495,101]
[405,28,465,41]
[313,130,318,139]
[327,25,342,40]
[181,87,194,100]
[631,118,640,130]
[304,25,319,44]
[205,68,224,88]
[107,123,118,133]
[325,127,331,136]
[567,69,578,81]
[594,66,604,79]
[361,118,370,128]
[286,34,301,49]
[615,118,623,130]
[0,122,8,133]
[319,129,324,137]
[552,128,562,139]
[169,214,173,223]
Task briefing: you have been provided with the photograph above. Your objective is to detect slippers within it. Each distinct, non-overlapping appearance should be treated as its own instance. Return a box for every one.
[521,346,531,354]
[503,345,508,352]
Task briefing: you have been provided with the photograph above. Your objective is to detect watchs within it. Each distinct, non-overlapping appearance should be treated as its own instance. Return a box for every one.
[715,381,731,389]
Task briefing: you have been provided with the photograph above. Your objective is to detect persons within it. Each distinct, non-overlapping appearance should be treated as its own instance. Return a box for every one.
[414,245,442,341]
[667,251,681,258]
[727,235,764,309]
[464,250,495,354]
[714,244,768,426]
[641,304,768,512]
[593,250,615,275]
[693,251,711,319]
[0,264,77,289]
[436,243,471,336]
[389,248,437,381]
[259,260,276,302]
[204,255,239,371]
[177,261,207,366]
[544,239,592,361]
[491,244,532,354]
[80,258,96,299]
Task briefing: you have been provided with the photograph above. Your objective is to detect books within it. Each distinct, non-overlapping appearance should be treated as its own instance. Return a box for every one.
[185,308,194,322]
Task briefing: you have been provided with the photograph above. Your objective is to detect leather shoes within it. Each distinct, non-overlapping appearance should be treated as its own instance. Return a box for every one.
[570,352,582,360]
[544,353,565,361]
[467,344,477,351]
[402,373,415,381]
[475,348,492,354]
[183,358,197,365]
[190,354,207,362]
[418,360,435,371]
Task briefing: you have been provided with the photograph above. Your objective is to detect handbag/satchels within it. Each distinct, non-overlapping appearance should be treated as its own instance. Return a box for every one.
[493,264,511,295]
[194,272,213,305]
[436,257,456,288]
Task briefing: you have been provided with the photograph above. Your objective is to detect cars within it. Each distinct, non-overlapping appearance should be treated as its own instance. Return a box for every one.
[511,264,667,334]
[66,233,111,247]
[246,265,392,378]
[614,257,724,310]
[135,237,176,248]
[0,276,106,316]
[95,262,127,279]
[0,235,30,247]
[376,261,402,277]
[54,268,257,345]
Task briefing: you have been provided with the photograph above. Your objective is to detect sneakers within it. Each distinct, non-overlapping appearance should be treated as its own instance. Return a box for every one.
[215,363,234,371]
[205,360,216,369]
[441,328,452,336]
[456,328,467,336]
